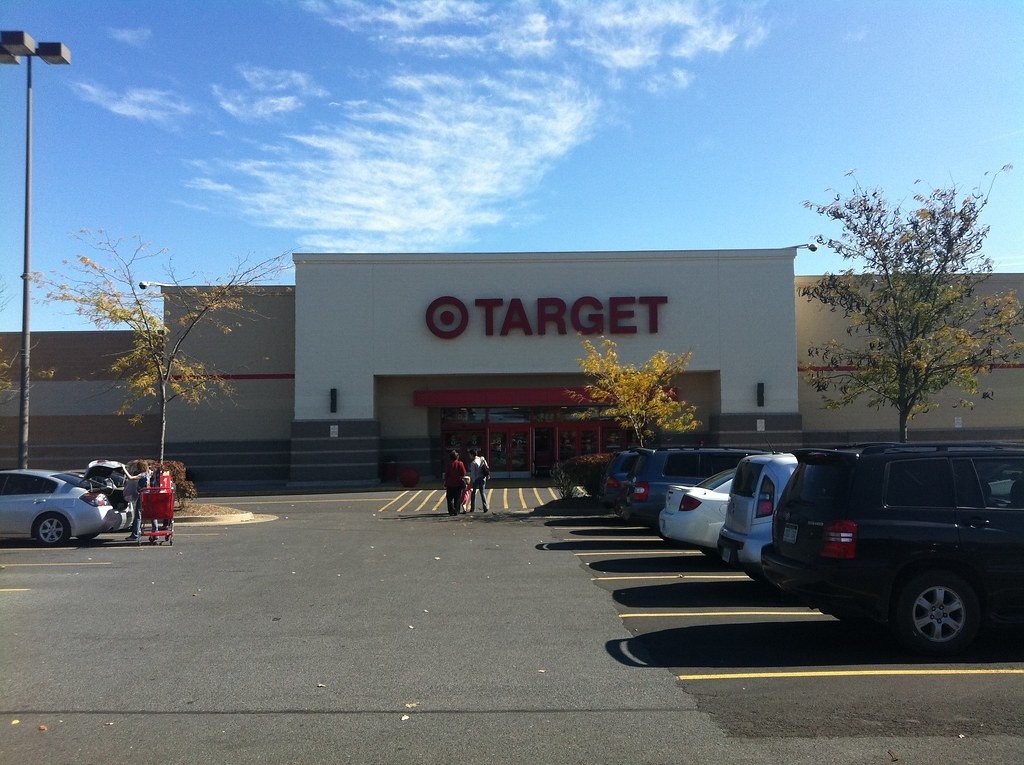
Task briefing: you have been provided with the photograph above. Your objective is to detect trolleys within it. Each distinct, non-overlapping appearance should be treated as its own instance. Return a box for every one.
[138,487,175,546]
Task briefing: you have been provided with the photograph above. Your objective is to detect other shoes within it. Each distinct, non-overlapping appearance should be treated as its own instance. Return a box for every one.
[125,535,140,541]
[484,508,488,513]
[149,535,160,541]
[469,509,474,513]
[165,534,169,541]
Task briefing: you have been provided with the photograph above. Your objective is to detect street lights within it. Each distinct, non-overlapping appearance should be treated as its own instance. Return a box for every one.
[0,30,71,470]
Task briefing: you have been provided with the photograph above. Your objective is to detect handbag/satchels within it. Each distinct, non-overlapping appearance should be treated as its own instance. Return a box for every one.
[123,477,139,502]
[481,456,490,476]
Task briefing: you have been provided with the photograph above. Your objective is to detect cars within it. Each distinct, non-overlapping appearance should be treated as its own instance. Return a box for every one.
[659,468,736,554]
[716,453,798,581]
[0,459,135,546]
[64,469,134,532]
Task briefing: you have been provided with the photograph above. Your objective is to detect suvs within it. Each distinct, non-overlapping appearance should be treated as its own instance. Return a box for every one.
[596,448,646,508]
[761,442,1024,657]
[616,445,770,522]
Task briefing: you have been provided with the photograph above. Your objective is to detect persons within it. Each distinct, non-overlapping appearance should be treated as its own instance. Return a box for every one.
[444,450,467,516]
[461,476,470,514]
[470,448,489,512]
[120,461,158,541]
